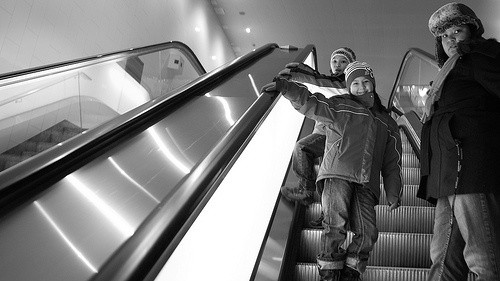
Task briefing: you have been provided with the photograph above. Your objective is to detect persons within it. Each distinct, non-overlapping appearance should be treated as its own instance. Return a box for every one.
[259,61,404,281]
[416,3,500,281]
[279,47,355,228]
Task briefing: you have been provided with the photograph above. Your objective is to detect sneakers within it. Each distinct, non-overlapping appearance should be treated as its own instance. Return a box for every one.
[281,187,315,205]
[309,214,324,228]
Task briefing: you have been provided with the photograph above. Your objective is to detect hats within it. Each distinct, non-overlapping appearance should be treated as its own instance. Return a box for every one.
[330,47,357,64]
[428,2,484,68]
[344,61,376,95]
